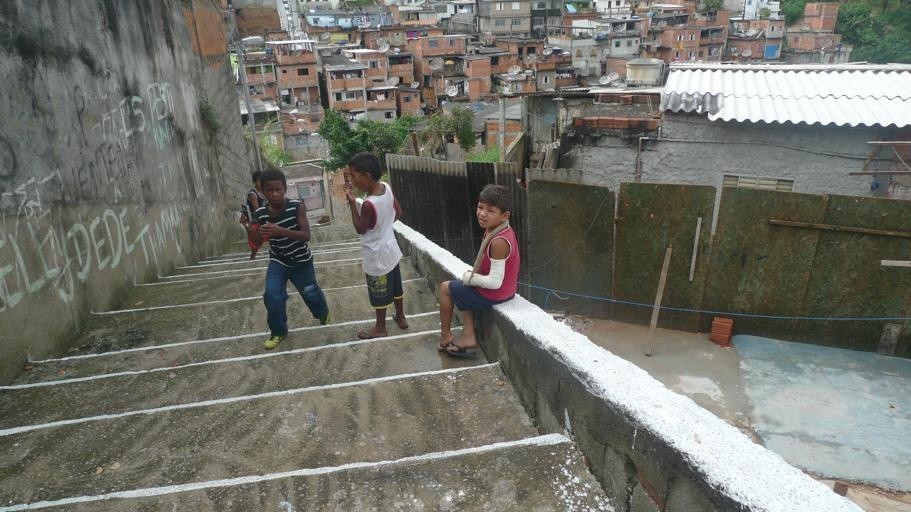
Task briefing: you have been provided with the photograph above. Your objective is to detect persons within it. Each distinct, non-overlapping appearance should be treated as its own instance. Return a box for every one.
[238,170,333,350]
[249,172,266,261]
[437,185,522,359]
[344,154,410,338]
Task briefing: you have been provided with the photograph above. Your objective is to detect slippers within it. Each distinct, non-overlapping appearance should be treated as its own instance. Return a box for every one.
[391,313,409,329]
[436,338,481,359]
[357,329,389,338]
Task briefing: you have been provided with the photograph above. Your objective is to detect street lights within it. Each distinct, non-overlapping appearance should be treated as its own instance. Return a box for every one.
[256,108,306,172]
[309,132,326,158]
[232,34,268,172]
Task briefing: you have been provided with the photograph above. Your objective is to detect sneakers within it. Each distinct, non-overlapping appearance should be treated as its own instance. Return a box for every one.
[320,311,333,326]
[262,330,289,350]
